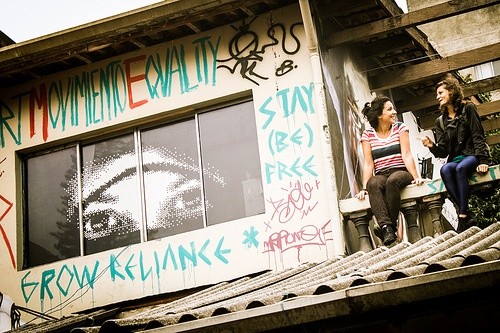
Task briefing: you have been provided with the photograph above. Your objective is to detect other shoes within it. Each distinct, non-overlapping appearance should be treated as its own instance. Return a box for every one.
[382,225,398,245]
[456,215,470,234]
[467,219,477,228]
[374,227,384,241]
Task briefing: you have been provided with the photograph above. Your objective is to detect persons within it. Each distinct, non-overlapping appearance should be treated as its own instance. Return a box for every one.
[355,94,429,243]
[416,74,492,234]
